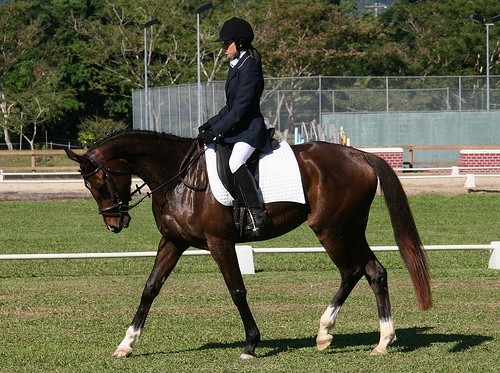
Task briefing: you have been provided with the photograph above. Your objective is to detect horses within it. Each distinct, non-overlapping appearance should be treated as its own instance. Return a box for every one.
[60,126,436,359]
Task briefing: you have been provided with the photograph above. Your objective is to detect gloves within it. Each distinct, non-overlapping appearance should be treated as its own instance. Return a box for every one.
[198,122,216,146]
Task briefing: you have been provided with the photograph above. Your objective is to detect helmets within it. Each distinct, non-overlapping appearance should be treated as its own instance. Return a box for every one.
[213,16,254,43]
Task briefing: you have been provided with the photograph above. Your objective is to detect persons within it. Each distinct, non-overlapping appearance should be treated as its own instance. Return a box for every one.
[199,17,272,234]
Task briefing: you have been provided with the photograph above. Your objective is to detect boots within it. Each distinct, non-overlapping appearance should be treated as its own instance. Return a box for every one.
[232,164,272,229]
